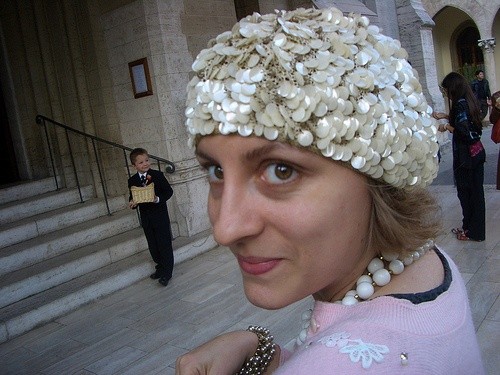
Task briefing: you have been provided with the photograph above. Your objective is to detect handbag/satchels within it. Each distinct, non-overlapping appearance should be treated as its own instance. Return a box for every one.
[468,140,487,166]
[491,119,500,143]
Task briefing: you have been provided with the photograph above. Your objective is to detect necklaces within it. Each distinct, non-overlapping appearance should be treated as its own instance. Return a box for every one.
[332,237,435,306]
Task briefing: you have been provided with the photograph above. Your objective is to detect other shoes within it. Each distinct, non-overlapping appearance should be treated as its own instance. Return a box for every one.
[150,269,160,278]
[452,228,467,234]
[457,233,482,242]
[159,276,169,285]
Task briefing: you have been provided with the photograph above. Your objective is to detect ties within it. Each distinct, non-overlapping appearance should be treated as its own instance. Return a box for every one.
[141,174,146,183]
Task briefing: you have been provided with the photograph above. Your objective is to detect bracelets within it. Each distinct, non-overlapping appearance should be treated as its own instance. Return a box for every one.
[235,325,275,375]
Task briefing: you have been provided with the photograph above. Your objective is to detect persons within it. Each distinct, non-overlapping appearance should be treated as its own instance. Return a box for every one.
[488,90,500,190]
[472,70,492,122]
[405,60,440,164]
[432,71,487,242]
[128,147,174,286]
[178,7,482,375]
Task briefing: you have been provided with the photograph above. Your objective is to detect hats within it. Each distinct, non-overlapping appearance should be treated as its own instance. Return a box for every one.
[183,6,441,193]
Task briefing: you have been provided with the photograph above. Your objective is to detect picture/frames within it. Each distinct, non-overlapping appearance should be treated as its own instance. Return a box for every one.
[127,57,153,99]
